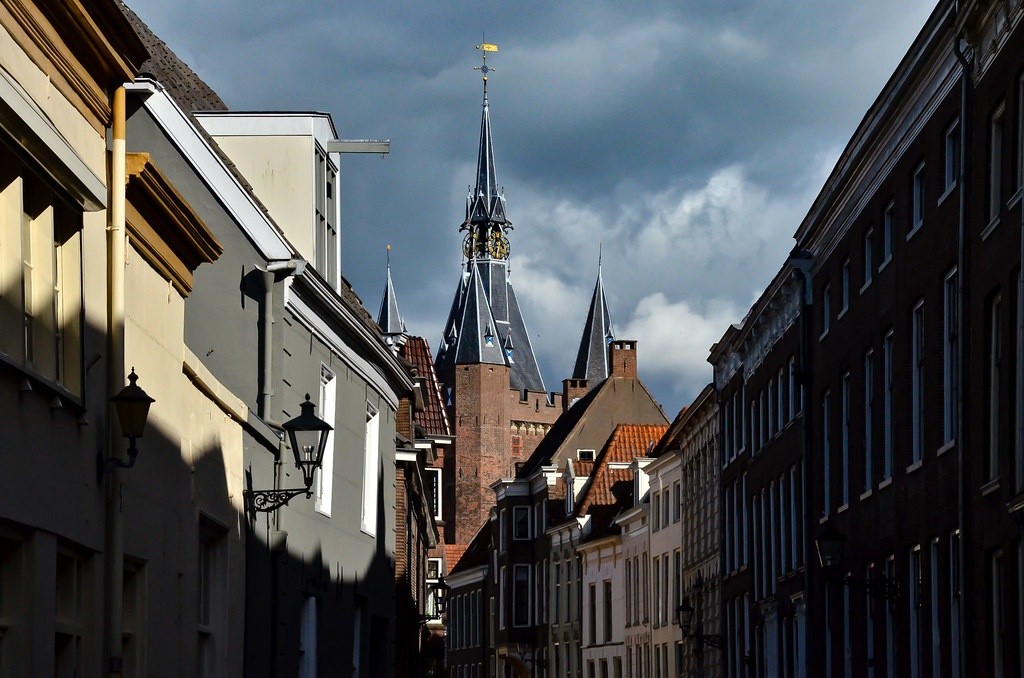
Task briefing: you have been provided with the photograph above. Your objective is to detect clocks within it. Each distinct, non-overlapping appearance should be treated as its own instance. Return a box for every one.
[461,232,482,259]
[487,232,508,258]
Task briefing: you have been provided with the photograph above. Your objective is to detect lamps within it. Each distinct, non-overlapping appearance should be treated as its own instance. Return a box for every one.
[675,590,722,651]
[419,567,453,621]
[242,390,337,526]
[813,514,903,612]
[96,366,155,487]
[517,639,550,669]
